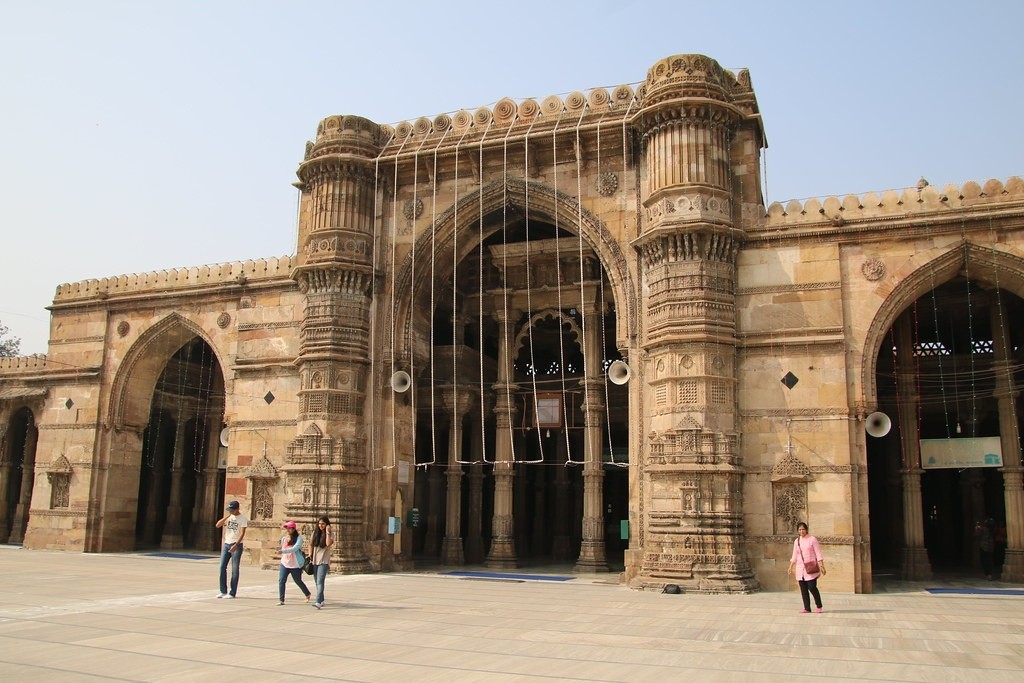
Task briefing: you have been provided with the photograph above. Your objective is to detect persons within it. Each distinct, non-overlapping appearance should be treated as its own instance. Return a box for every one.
[787,522,826,613]
[214,501,248,598]
[308,516,334,609]
[275,521,311,605]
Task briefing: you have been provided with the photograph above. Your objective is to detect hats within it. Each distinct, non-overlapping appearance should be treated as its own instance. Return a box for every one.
[225,501,239,512]
[284,521,296,529]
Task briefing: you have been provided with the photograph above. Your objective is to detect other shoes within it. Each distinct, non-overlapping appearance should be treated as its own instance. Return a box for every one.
[276,601,284,606]
[216,592,227,597]
[304,594,311,603]
[312,601,325,606]
[222,594,234,599]
[312,603,321,609]
[815,608,822,613]
[800,609,810,613]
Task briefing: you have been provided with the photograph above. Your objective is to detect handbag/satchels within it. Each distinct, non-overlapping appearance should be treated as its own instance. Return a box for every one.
[303,557,314,575]
[804,561,819,574]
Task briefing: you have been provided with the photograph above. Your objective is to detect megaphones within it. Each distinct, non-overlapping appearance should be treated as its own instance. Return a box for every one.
[391,371,411,392]
[220,428,229,447]
[865,412,891,437]
[608,361,631,385]
